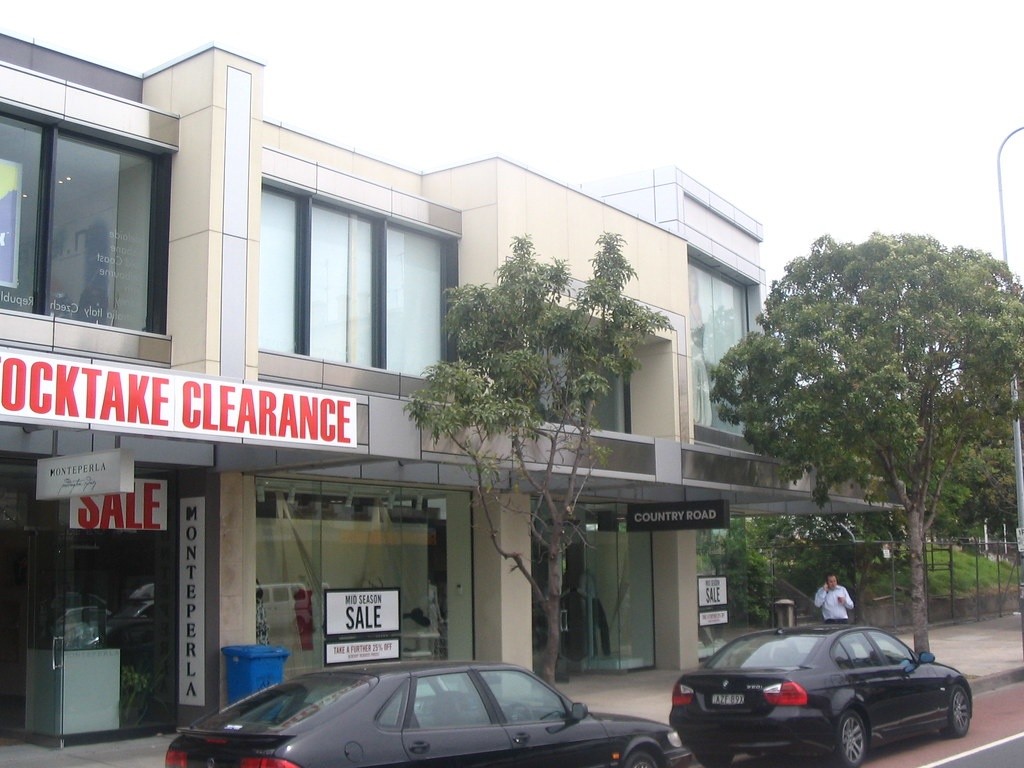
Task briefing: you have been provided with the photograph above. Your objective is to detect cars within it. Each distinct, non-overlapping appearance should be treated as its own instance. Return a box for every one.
[163,658,694,768]
[666,626,975,768]
[55,605,113,644]
[74,600,158,658]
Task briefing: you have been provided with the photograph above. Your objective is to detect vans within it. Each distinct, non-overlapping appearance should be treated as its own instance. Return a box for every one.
[255,582,311,636]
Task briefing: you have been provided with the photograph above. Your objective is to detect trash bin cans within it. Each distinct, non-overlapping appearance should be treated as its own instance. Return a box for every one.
[219,643,291,704]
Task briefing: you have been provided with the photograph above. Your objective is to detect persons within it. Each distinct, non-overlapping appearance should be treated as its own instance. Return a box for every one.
[814,573,853,624]
[255,577,270,644]
[293,575,314,651]
[421,577,443,653]
